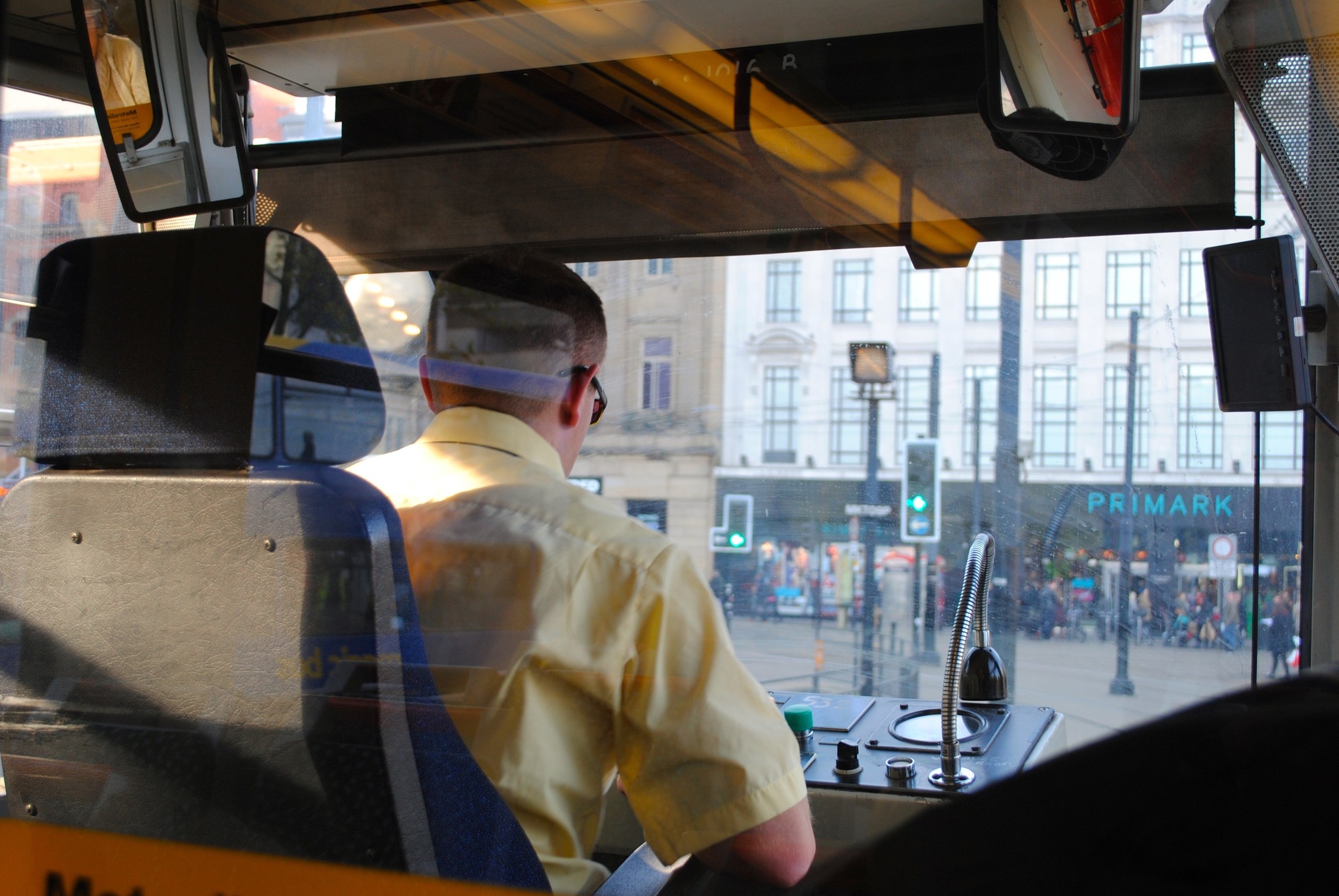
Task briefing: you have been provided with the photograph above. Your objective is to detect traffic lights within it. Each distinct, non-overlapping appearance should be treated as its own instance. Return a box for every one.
[900,437,940,542]
[723,494,752,553]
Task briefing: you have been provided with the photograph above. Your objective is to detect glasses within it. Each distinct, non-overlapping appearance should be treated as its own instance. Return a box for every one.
[559,365,608,427]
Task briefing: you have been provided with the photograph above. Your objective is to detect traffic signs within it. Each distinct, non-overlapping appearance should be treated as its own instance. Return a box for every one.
[845,504,892,518]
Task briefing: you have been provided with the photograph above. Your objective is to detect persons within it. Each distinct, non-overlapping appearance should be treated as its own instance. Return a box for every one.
[705,566,779,622]
[344,249,817,893]
[1125,582,1303,679]
[988,578,1081,637]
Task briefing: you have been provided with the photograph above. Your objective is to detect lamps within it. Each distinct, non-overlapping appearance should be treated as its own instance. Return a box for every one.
[927,532,1009,786]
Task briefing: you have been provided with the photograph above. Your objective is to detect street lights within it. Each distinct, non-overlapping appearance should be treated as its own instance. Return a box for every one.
[850,339,892,697]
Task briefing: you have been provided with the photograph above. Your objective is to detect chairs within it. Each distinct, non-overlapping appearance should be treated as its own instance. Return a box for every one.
[0,226,555,890]
